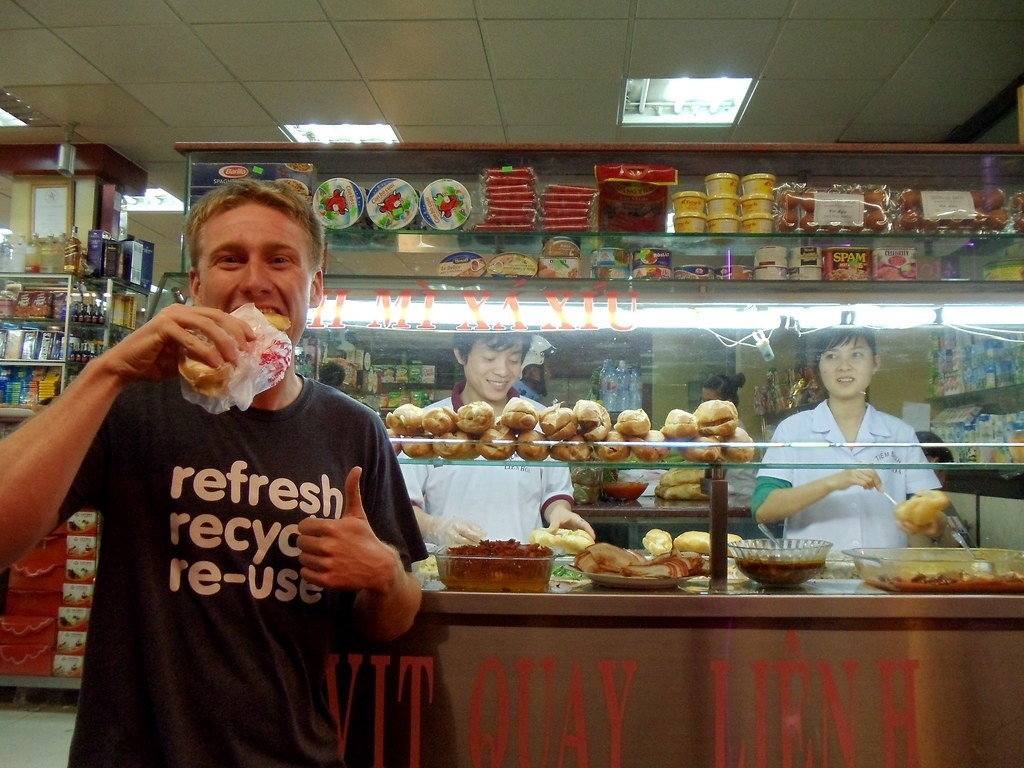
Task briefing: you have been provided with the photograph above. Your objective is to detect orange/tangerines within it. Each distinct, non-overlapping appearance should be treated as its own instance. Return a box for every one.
[776,183,1024,231]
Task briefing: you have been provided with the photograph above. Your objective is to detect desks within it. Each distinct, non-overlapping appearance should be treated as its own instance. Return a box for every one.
[572,505,753,549]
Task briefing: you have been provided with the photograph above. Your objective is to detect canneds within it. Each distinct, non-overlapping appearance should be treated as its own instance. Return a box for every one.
[440,236,1024,281]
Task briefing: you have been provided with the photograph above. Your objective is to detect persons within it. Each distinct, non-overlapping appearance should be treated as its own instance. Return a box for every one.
[905,431,965,549]
[749,329,948,564]
[0,181,431,768]
[512,334,559,407]
[396,332,596,549]
[698,373,746,433]
[318,360,346,387]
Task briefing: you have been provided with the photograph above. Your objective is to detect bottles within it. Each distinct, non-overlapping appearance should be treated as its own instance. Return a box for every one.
[68,343,103,363]
[0,225,87,276]
[170,287,186,304]
[61,301,106,324]
[586,358,642,420]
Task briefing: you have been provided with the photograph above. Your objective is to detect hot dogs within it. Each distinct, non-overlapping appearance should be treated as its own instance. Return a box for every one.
[382,397,953,579]
[178,308,292,397]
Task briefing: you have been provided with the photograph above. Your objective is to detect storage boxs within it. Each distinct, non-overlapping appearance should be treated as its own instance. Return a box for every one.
[371,364,436,408]
[88,229,117,277]
[117,238,156,288]
[0,509,100,675]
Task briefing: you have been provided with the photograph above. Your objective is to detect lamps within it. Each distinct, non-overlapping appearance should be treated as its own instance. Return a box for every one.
[56,120,77,177]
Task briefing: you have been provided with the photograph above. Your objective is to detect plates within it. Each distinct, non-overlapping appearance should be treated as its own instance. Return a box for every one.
[568,563,691,588]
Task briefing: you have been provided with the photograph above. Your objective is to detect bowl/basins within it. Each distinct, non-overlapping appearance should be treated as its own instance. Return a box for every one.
[729,538,834,587]
[1006,429,1024,463]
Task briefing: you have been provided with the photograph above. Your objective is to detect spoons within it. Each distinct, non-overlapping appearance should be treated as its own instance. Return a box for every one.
[950,531,993,575]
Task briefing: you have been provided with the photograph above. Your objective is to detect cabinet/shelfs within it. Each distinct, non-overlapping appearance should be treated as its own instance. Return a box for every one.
[0,272,151,412]
[176,140,1022,767]
